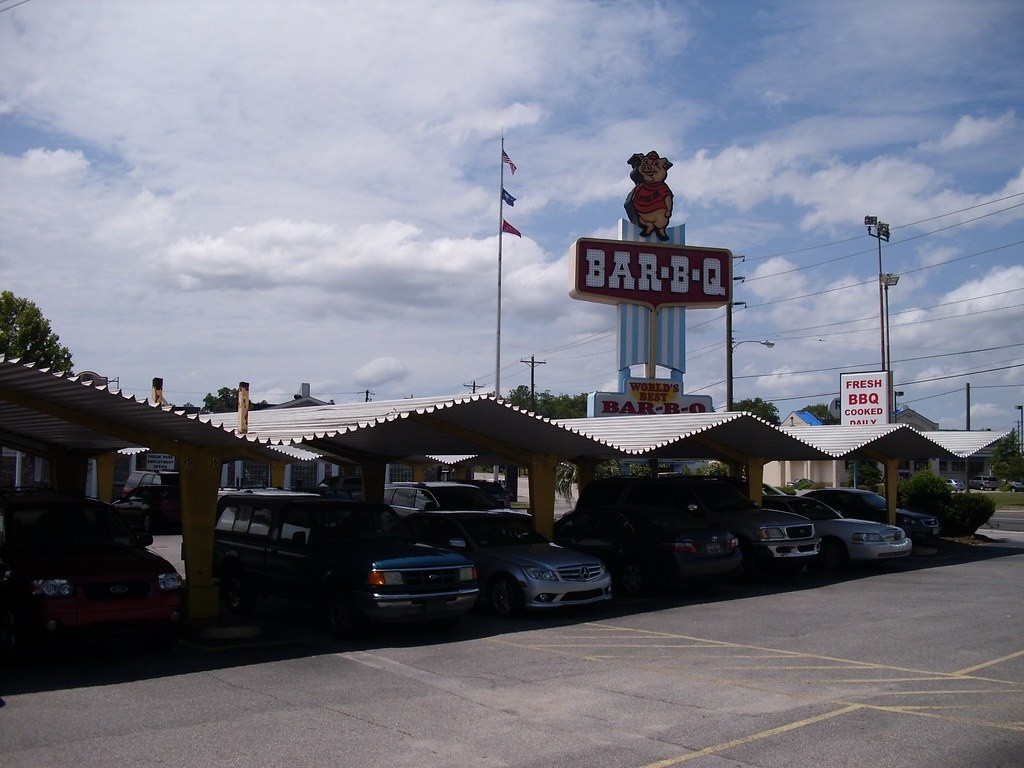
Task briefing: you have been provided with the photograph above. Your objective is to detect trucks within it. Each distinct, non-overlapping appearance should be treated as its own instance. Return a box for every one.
[121,470,179,498]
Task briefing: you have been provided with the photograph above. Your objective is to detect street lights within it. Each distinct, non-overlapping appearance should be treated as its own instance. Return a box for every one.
[881,273,899,422]
[726,340,775,411]
[865,215,890,371]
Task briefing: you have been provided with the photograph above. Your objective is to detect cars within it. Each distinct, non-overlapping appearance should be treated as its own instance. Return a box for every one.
[401,510,613,619]
[553,505,742,599]
[0,485,187,667]
[946,479,965,491]
[1008,480,1024,492]
[110,485,181,533]
[762,490,940,571]
[969,475,998,490]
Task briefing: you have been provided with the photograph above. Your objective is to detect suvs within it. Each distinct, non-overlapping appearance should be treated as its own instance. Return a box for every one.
[574,475,822,582]
[182,476,533,639]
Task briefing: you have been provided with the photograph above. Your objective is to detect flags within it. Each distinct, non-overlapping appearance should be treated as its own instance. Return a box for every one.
[503,150,517,175]
[503,220,521,239]
[502,188,517,207]
